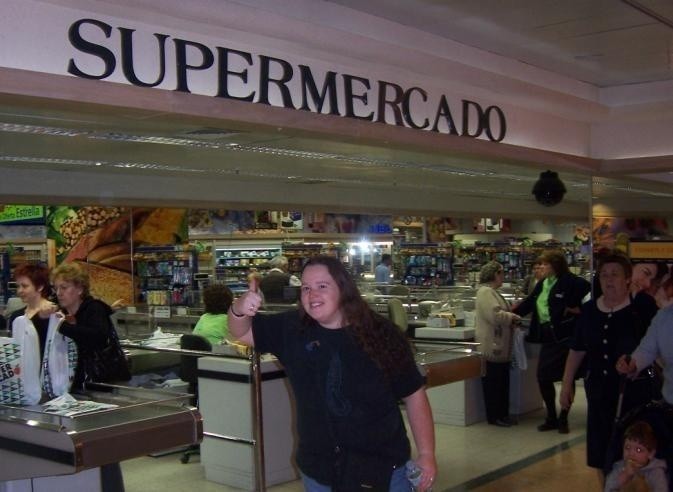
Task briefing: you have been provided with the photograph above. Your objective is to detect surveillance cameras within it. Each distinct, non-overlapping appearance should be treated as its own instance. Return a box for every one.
[531,174,567,207]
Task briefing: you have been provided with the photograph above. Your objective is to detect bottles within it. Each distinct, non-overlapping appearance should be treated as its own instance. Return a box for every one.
[405,460,434,492]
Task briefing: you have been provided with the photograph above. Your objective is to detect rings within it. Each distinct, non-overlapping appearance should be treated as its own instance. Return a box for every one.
[430,478,434,482]
[249,304,254,312]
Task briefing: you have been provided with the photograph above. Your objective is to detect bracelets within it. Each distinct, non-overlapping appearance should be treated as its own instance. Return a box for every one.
[230,306,245,318]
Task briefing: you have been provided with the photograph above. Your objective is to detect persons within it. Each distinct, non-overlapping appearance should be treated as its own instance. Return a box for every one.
[257,255,301,308]
[193,284,234,344]
[559,253,673,492]
[226,256,437,492]
[376,253,393,285]
[512,249,591,431]
[527,261,543,296]
[9,260,75,406]
[39,262,133,492]
[475,260,523,427]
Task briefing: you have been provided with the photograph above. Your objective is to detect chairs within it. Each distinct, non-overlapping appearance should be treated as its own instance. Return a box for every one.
[180,335,212,464]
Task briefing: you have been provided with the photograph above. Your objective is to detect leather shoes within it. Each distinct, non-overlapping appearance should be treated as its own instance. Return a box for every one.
[491,417,517,427]
[538,418,569,433]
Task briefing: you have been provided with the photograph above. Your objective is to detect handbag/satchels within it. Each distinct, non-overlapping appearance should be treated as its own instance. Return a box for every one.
[511,329,526,369]
[88,345,119,381]
[0,312,78,406]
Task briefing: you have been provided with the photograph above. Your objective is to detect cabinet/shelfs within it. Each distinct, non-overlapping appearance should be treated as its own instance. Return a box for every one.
[132,240,590,306]
